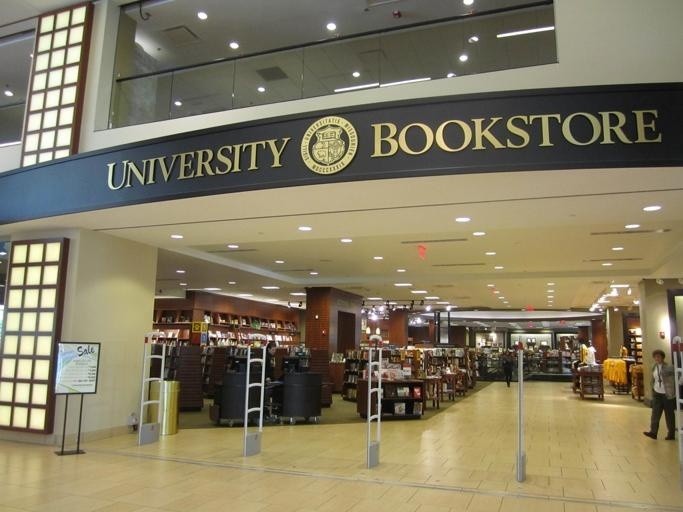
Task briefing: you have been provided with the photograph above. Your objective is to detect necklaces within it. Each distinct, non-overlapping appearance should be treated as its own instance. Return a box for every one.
[656,364,662,387]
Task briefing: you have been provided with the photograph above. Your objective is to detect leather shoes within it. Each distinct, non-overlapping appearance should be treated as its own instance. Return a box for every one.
[644,431,657,439]
[665,435,675,440]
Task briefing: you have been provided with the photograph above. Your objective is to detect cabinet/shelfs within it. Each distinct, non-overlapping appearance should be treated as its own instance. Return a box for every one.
[571,329,645,402]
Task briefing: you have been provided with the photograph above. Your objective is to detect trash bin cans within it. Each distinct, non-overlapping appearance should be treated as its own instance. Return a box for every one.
[147,380,179,435]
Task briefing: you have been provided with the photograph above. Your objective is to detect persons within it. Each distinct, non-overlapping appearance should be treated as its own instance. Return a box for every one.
[265,342,276,380]
[502,350,514,387]
[642,350,677,440]
